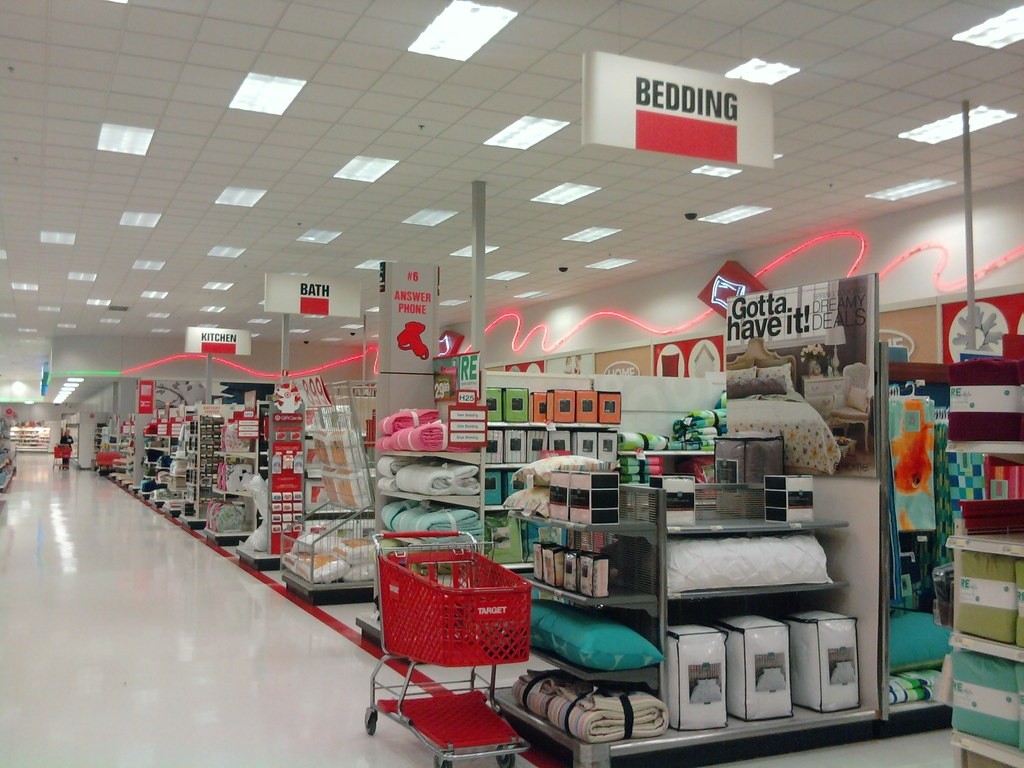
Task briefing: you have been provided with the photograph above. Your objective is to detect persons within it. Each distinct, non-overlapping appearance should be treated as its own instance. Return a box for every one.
[60,430,74,470]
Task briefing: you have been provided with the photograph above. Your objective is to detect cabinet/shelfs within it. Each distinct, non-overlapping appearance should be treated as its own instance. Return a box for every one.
[0,362,1024,768]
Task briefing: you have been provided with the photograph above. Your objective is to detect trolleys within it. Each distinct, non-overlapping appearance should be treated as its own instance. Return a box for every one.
[365,530,533,768]
[53,445,72,470]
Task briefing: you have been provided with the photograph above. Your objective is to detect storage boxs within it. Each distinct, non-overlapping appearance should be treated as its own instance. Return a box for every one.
[648,474,695,510]
[541,545,563,586]
[485,470,503,505]
[718,611,795,722]
[578,390,599,424]
[505,469,528,503]
[1015,561,1024,651]
[527,430,549,462]
[486,429,504,464]
[574,431,598,459]
[545,388,576,423]
[762,474,816,524]
[547,468,568,520]
[563,548,582,593]
[713,431,786,514]
[947,651,1024,748]
[505,427,527,463]
[532,540,557,582]
[486,386,501,422]
[600,430,620,459]
[597,389,624,424]
[572,469,621,524]
[956,551,1016,644]
[531,391,547,422]
[667,621,728,735]
[503,385,530,422]
[579,552,610,599]
[549,430,572,458]
[782,610,862,716]
